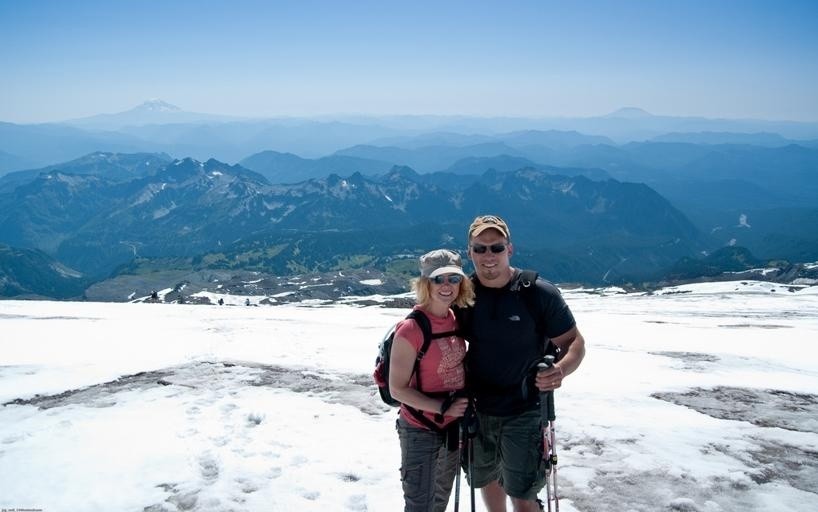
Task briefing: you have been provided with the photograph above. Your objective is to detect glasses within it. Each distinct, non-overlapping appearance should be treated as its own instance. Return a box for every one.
[469,243,505,254]
[429,275,462,284]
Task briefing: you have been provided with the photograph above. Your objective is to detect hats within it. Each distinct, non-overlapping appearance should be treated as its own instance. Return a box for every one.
[468,215,511,239]
[419,249,464,278]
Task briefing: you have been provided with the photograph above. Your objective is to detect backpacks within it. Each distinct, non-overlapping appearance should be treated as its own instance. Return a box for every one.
[373,308,432,408]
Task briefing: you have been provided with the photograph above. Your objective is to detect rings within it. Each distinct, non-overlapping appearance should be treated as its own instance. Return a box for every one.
[552,382,556,386]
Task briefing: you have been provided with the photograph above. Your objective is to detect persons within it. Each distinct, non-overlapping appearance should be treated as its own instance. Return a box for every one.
[388,248,478,511]
[450,215,586,512]
